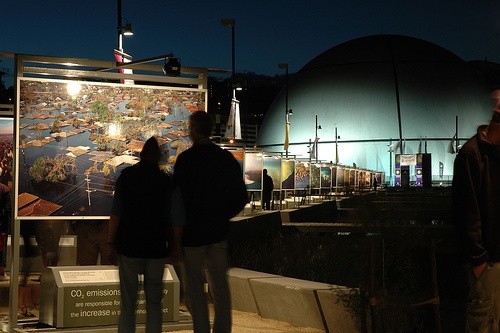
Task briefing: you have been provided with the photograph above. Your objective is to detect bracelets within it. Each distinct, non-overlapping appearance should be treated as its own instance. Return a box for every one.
[106,241,118,244]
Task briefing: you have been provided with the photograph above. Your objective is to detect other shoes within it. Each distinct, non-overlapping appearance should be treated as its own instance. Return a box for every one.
[21,311,35,317]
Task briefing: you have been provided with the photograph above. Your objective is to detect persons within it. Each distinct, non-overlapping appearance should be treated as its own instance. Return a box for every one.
[261,169,273,210]
[452,90,500,333]
[106,136,186,333]
[0,133,119,320]
[174,112,248,333]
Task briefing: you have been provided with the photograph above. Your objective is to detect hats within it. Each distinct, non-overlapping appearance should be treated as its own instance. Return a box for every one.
[492,109,500,123]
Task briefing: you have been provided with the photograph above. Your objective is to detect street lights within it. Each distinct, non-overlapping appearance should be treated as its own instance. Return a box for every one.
[277,64,293,158]
[315,114,322,161]
[335,127,341,163]
[219,18,243,142]
[118,1,134,51]
